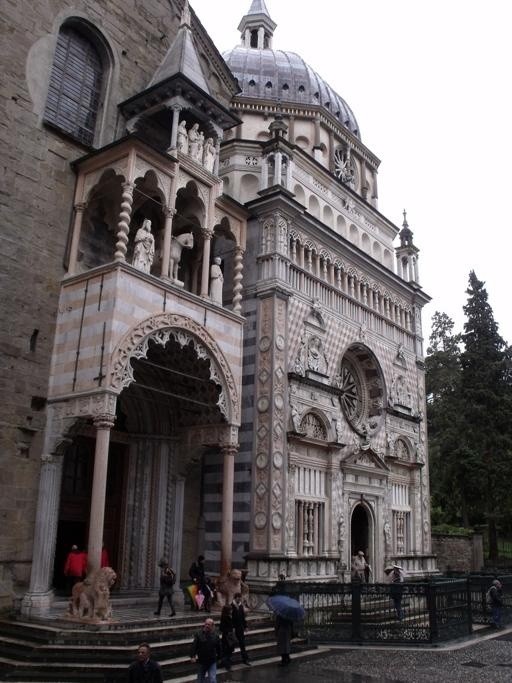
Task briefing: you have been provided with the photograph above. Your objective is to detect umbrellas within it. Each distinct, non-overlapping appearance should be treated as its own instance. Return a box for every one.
[267,595,307,622]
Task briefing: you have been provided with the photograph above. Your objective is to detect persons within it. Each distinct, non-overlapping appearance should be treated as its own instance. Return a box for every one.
[210,255,224,305]
[190,617,221,682]
[352,550,366,581]
[188,123,199,161]
[101,541,109,567]
[125,641,163,681]
[274,615,294,666]
[197,130,204,142]
[490,579,504,629]
[389,565,404,620]
[154,542,252,669]
[177,119,189,153]
[203,137,215,173]
[132,218,156,274]
[64,544,87,596]
